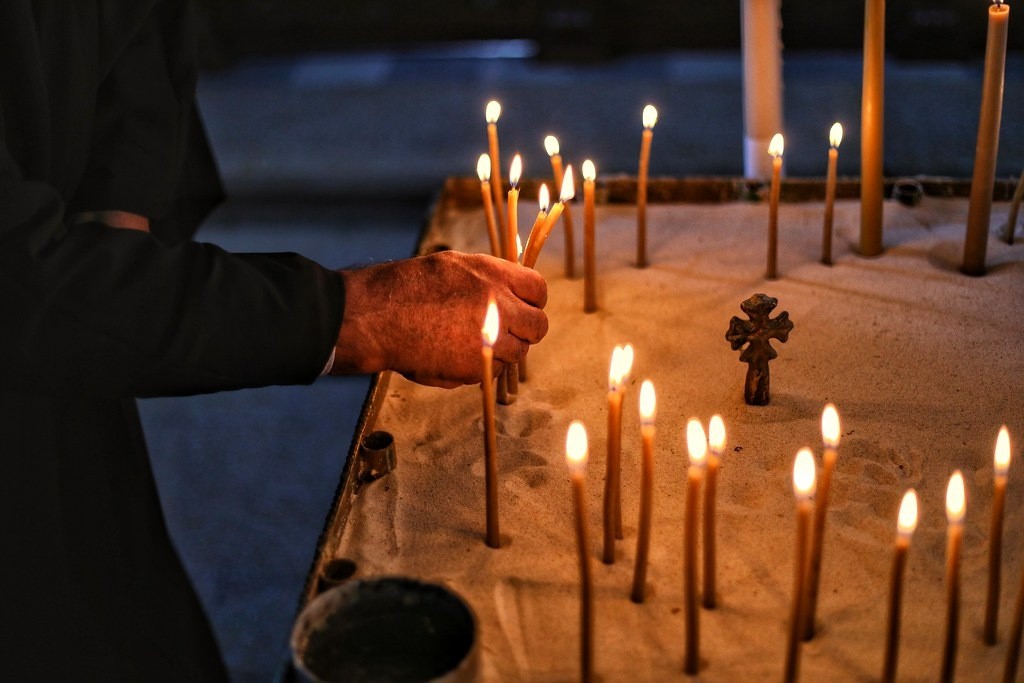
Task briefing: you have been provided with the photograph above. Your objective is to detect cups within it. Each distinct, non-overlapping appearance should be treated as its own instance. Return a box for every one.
[290,574,478,683]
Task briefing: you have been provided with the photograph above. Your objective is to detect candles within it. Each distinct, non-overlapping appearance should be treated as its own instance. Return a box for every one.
[474,1,1024,683]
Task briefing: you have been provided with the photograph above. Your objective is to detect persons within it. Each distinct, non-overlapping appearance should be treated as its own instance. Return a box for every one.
[0,1,551,682]
